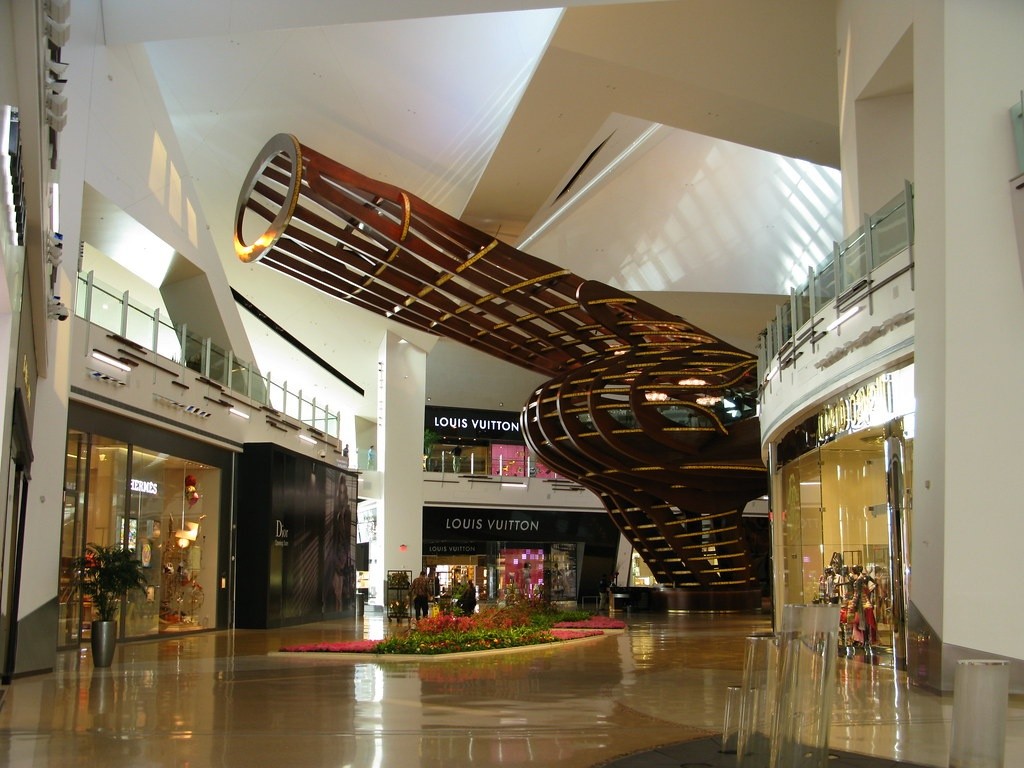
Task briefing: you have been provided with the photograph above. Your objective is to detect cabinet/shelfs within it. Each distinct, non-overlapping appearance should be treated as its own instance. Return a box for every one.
[388,570,413,624]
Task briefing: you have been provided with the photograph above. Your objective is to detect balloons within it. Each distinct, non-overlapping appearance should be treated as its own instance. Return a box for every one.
[184,475,198,509]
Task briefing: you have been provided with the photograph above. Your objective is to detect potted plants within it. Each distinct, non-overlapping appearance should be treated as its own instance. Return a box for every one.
[70,540,150,667]
[423,429,443,472]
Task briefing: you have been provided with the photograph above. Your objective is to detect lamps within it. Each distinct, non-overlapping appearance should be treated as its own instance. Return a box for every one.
[502,484,528,488]
[299,434,318,445]
[175,520,199,550]
[92,352,132,372]
[229,408,250,419]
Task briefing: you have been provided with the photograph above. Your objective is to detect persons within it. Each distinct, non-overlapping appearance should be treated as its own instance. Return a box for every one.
[508,568,531,594]
[368,445,375,470]
[343,444,349,457]
[451,445,462,474]
[818,563,879,657]
[332,475,351,614]
[412,571,432,621]
